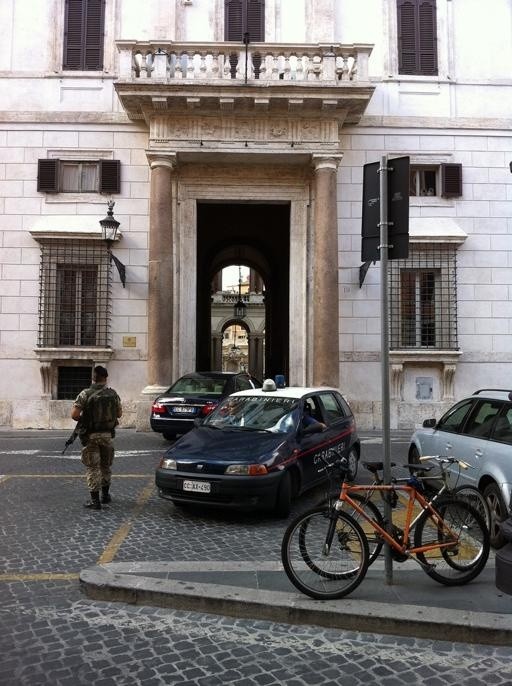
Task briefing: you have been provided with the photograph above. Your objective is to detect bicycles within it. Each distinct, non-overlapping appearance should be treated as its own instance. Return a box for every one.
[279,452,492,601]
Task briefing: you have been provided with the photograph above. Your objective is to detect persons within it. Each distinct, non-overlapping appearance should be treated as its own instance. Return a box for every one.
[263,400,327,436]
[209,398,254,426]
[71,365,123,511]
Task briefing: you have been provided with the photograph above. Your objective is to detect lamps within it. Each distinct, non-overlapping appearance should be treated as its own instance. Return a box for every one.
[233,267,246,319]
[99,200,121,253]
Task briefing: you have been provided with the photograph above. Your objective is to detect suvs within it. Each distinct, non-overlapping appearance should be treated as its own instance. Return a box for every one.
[403,387,512,551]
[154,376,362,522]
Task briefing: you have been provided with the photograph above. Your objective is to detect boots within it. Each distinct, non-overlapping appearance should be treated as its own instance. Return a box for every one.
[83,491,101,509]
[101,485,110,504]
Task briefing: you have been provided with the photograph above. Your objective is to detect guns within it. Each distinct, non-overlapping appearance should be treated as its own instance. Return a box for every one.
[62,412,83,455]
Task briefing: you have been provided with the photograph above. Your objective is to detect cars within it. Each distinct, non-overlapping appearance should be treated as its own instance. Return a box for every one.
[149,371,265,442]
